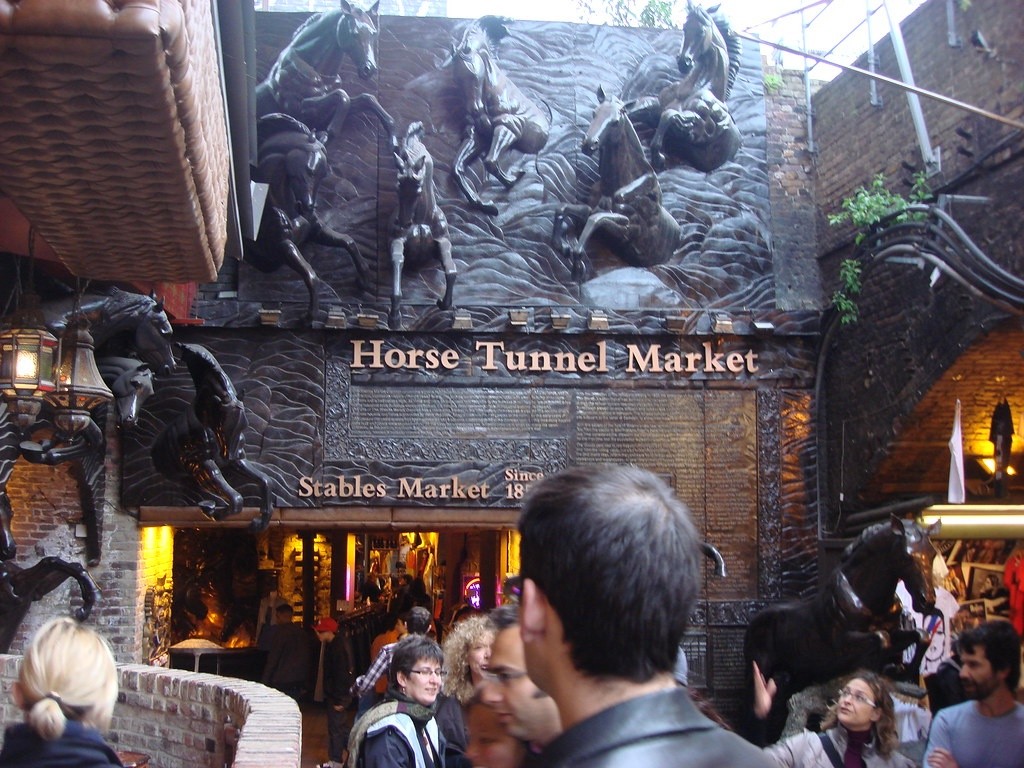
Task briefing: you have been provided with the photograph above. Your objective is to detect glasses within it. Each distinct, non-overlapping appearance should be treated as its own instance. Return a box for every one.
[406,669,447,678]
[839,687,878,708]
[479,664,529,688]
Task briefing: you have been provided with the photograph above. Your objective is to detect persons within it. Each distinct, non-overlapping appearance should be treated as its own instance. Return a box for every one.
[261,572,734,768]
[922,620,1024,768]
[0,616,123,768]
[519,464,782,768]
[753,659,918,768]
[980,574,1010,617]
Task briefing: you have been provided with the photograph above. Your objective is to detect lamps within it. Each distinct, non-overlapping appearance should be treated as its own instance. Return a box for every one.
[965,456,1016,497]
[0,309,57,434]
[356,314,380,330]
[665,316,687,333]
[451,309,475,332]
[42,319,115,446]
[507,309,529,325]
[752,321,776,335]
[711,314,734,335]
[550,314,572,330]
[970,29,997,57]
[325,312,347,330]
[586,310,610,331]
[258,310,282,326]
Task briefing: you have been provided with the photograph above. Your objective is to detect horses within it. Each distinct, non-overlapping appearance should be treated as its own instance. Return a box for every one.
[233,0,746,334]
[0,288,279,654]
[741,511,940,716]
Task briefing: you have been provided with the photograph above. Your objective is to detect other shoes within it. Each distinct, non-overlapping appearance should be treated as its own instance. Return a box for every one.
[316,761,343,768]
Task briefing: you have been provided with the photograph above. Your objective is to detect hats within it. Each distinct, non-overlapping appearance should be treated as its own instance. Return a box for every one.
[311,616,338,634]
[673,644,689,687]
